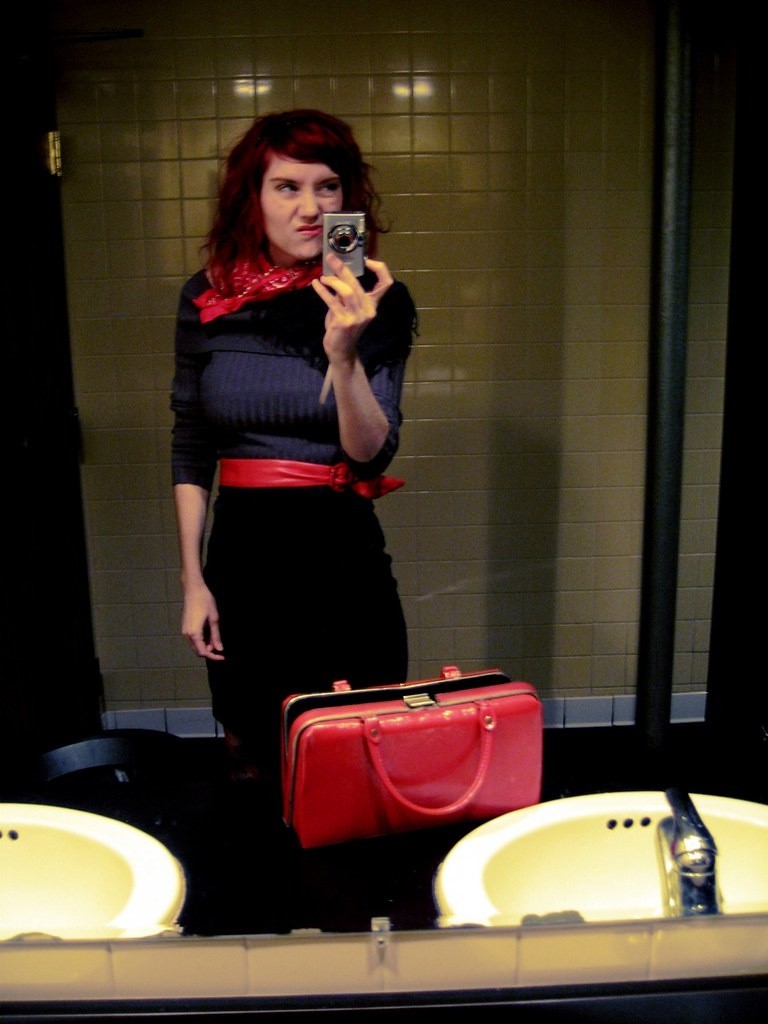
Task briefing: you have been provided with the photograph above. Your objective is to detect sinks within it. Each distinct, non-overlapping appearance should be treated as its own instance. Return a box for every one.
[435,788,768,928]
[0,802,186,940]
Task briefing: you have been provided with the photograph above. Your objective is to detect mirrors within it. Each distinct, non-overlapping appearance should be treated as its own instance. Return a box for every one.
[0,0,768,1024]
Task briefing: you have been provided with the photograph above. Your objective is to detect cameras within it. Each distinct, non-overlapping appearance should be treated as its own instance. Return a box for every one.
[322,210,366,279]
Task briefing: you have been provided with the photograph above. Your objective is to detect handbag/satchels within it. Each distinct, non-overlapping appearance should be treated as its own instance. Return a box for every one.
[281,664,544,850]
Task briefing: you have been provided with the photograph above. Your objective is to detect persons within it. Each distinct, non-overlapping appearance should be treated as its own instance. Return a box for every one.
[167,108,421,777]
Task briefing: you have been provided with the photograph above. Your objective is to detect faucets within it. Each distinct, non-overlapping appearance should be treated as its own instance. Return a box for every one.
[655,775,724,917]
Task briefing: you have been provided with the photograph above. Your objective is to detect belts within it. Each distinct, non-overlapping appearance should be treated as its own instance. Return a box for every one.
[219,457,406,500]
